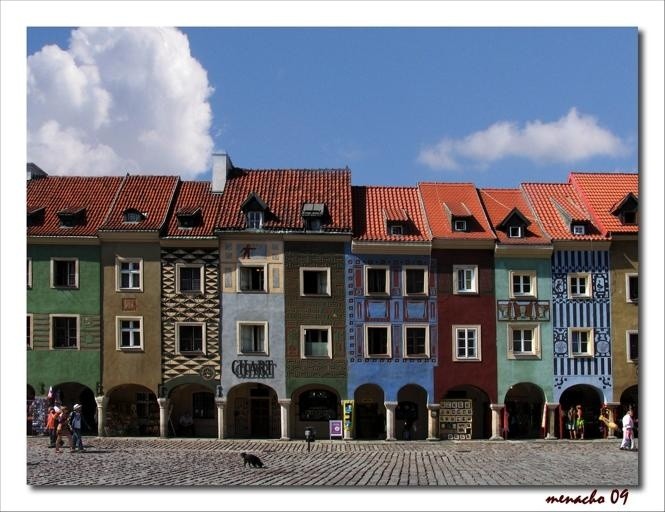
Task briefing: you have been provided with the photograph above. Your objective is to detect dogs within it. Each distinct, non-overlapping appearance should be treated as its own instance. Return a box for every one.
[240,452,264,469]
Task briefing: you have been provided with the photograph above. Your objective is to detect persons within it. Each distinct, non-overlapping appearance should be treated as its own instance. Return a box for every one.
[620,407,638,451]
[46,399,87,454]
[568,406,578,440]
[574,404,585,440]
[179,410,194,424]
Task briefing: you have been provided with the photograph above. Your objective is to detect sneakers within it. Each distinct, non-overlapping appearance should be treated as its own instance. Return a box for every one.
[66,444,77,453]
[55,451,63,453]
[48,441,64,448]
[78,450,86,452]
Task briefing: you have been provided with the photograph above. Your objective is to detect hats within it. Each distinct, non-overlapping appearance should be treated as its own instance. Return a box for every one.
[73,403,81,410]
